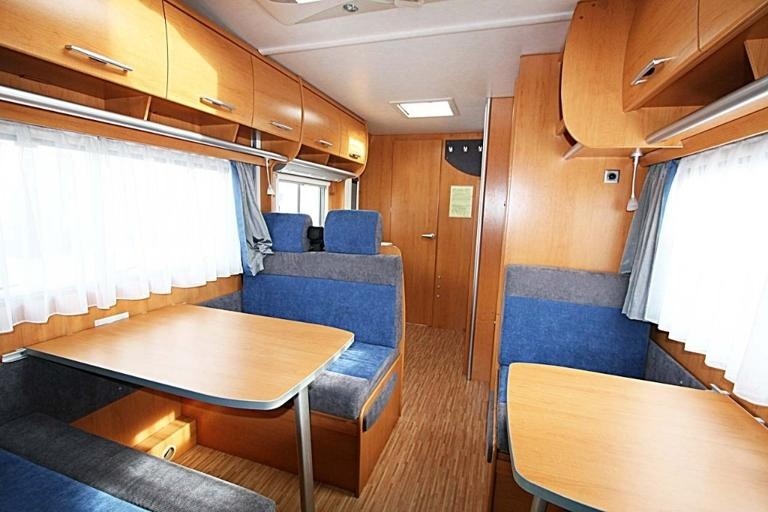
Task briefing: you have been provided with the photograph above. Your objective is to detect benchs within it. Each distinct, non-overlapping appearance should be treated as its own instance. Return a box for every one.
[492,264,658,509]
[1,414,276,511]
[182,212,411,496]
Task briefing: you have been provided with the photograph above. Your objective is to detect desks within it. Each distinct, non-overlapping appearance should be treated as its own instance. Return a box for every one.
[506,358,767,512]
[30,302,357,510]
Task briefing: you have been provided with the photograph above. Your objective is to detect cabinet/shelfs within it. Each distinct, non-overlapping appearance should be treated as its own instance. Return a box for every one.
[624,1,768,147]
[248,48,304,173]
[273,75,339,180]
[322,103,369,183]
[160,1,254,162]
[2,1,167,141]
